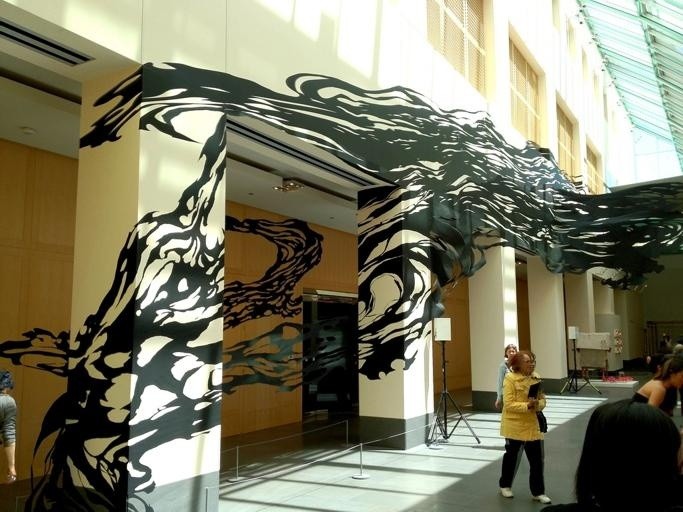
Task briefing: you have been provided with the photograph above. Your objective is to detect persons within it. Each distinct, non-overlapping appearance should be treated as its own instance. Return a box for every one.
[495,344,552,504]
[0,370,17,483]
[540,344,683,512]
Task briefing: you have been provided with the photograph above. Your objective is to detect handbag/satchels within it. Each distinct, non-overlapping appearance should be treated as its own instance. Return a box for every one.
[535,410,549,433]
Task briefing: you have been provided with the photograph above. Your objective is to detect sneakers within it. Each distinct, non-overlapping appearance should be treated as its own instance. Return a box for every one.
[531,493,552,504]
[499,487,514,498]
